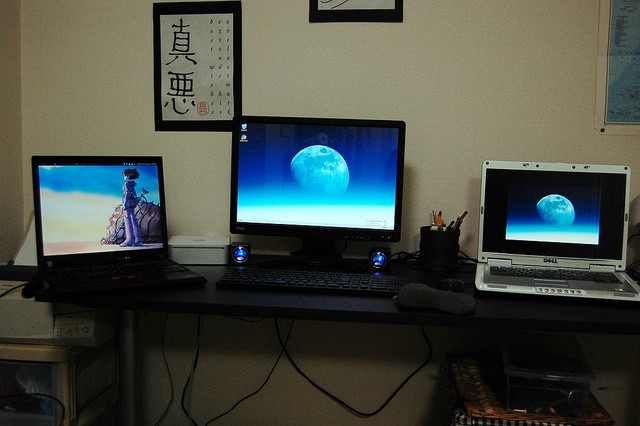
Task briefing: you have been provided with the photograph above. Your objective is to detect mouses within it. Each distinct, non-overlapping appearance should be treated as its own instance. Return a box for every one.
[441,278,465,294]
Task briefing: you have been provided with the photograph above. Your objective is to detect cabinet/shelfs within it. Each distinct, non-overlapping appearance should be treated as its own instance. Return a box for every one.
[1,336,120,424]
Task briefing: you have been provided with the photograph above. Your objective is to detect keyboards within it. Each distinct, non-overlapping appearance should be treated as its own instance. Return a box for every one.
[216,266,408,297]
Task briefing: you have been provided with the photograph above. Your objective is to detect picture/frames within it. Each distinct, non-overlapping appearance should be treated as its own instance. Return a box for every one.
[153,2,242,132]
[308,0,404,24]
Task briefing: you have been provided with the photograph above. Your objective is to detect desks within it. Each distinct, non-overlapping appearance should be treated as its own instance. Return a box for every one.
[35,256,639,425]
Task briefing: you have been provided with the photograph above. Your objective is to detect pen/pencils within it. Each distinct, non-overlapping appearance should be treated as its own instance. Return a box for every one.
[437,211,442,224]
[432,210,437,225]
[455,211,468,229]
[446,221,454,229]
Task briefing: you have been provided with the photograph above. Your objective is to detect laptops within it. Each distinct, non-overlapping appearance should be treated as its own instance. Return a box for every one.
[31,155,208,301]
[474,160,640,302]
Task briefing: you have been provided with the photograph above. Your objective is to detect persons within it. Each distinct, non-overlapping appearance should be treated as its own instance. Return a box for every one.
[123,170,141,246]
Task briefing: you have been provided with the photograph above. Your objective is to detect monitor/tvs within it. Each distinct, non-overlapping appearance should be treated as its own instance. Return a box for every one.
[229,115,407,269]
